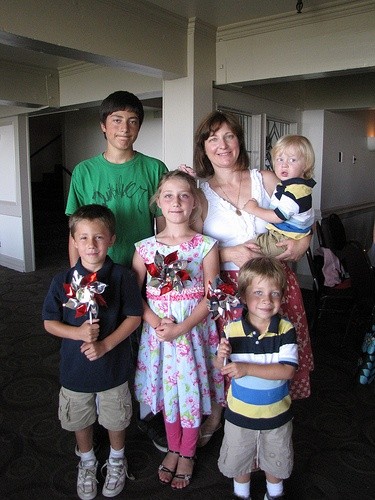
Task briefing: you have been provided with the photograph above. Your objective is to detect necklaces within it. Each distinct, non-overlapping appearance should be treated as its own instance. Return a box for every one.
[214,173,242,217]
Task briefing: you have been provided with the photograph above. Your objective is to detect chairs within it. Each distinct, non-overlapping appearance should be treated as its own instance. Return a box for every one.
[320,213,375,295]
[306,246,359,339]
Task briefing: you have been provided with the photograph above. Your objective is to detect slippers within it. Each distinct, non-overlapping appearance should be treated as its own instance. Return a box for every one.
[197,420,222,447]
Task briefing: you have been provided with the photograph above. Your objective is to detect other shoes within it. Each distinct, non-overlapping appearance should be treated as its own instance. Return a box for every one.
[233,493,251,500]
[264,493,291,500]
[137,418,169,453]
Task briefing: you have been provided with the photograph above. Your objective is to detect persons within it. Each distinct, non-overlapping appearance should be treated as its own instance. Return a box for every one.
[42,204,143,500]
[242,135,317,260]
[132,170,219,491]
[65,91,169,454]
[194,111,315,472]
[213,257,299,500]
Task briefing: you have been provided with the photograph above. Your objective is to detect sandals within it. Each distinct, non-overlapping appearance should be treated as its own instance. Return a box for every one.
[157,450,179,485]
[172,455,194,491]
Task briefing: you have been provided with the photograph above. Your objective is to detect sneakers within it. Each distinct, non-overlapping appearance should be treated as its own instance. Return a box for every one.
[102,459,128,497]
[75,459,98,499]
